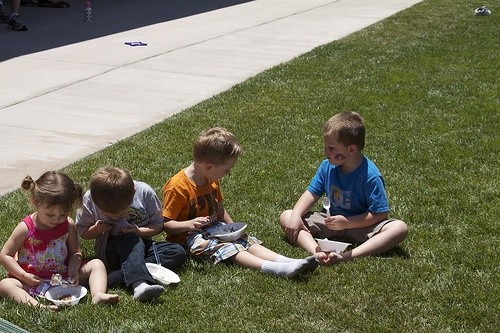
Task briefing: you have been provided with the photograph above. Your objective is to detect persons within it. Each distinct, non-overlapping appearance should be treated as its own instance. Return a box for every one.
[160,126,319,278]
[0,0,29,33]
[1,169,119,311]
[278,108,409,267]
[73,166,186,301]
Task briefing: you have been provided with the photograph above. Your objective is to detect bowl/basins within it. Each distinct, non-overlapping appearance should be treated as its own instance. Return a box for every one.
[316,238,352,255]
[45,284,87,306]
[208,222,247,241]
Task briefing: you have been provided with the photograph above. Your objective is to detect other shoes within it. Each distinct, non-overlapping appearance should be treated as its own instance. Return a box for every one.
[37,0,52,8]
[53,2,64,8]
[474,7,491,16]
[20,0,35,7]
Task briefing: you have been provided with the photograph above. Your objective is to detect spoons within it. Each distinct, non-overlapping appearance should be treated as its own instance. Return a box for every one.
[323,197,331,217]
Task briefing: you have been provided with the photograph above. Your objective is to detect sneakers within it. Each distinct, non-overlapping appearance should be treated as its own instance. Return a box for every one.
[0,6,12,23]
[9,16,28,31]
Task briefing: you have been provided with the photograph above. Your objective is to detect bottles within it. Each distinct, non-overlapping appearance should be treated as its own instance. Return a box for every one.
[84,3,92,22]
